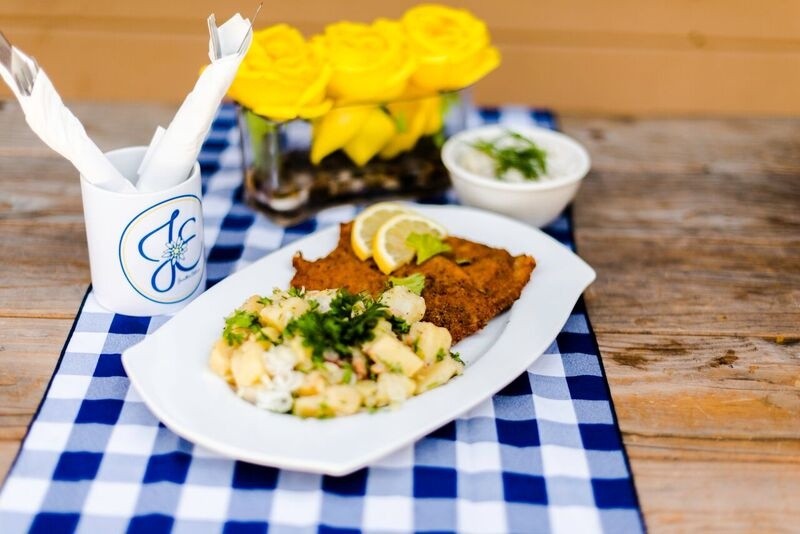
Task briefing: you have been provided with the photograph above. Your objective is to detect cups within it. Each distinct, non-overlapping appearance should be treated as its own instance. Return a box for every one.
[80,146,207,317]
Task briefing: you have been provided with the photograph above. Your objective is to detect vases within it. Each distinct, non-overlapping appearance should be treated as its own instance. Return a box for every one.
[233,90,476,228]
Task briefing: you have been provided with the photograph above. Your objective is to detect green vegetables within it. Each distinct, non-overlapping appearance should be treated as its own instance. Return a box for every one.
[468,131,547,180]
[406,233,456,266]
[389,274,426,297]
[220,285,467,415]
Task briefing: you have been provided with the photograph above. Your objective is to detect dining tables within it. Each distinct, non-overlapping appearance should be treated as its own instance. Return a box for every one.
[0,101,800,534]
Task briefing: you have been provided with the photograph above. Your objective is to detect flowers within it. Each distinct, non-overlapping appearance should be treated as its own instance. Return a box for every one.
[199,3,502,167]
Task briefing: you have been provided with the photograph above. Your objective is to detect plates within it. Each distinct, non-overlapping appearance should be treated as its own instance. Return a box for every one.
[122,202,598,479]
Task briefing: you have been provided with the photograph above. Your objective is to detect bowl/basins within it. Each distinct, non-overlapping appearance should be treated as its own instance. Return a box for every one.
[441,123,591,228]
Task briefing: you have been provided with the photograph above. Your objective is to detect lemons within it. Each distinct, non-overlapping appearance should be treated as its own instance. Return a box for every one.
[351,201,421,261]
[371,214,448,275]
